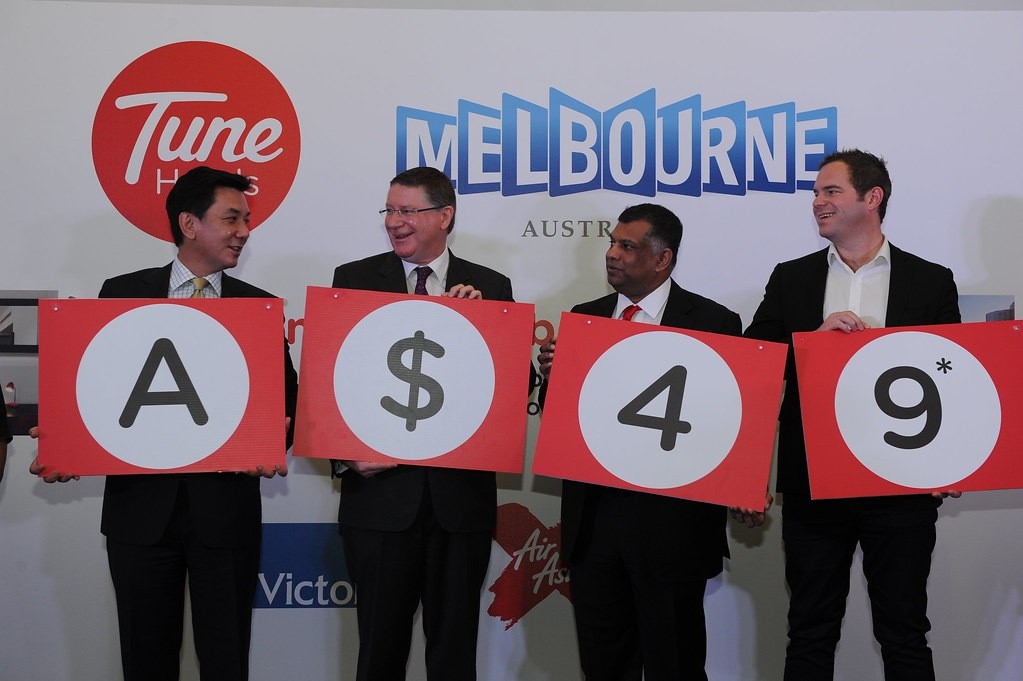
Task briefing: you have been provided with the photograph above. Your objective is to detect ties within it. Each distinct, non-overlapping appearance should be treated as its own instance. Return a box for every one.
[190,278,210,298]
[622,305,643,321]
[413,266,434,295]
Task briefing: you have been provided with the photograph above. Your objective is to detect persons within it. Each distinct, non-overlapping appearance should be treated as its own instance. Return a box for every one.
[537,203,773,681]
[28,166,299,681]
[744,149,965,681]
[331,167,536,681]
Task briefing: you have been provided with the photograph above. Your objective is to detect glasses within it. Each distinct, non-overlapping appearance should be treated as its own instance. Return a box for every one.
[379,206,445,220]
